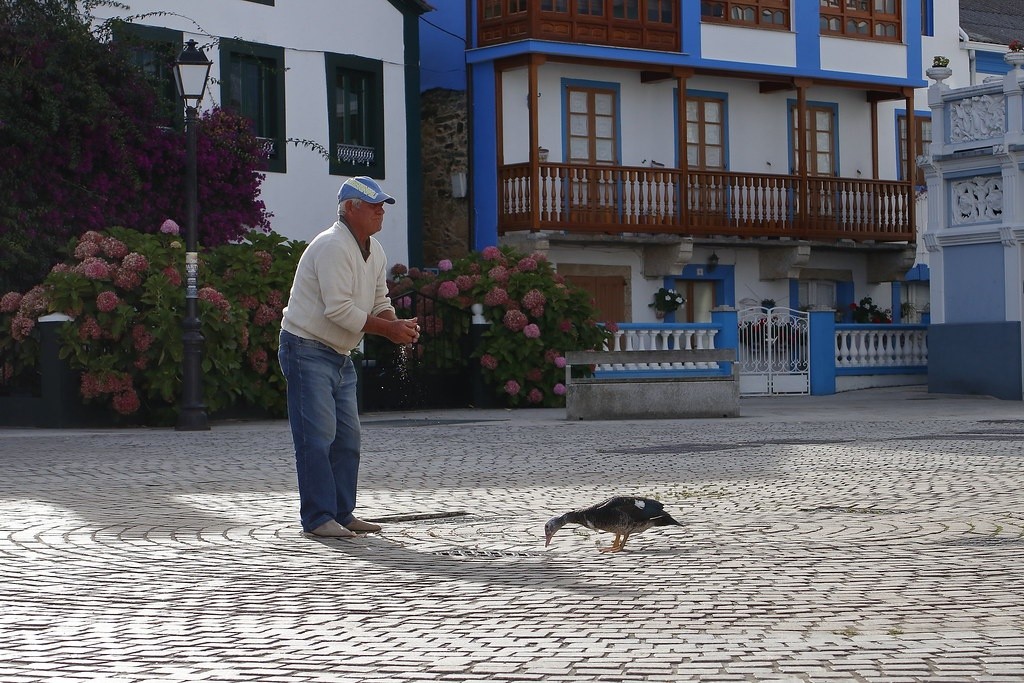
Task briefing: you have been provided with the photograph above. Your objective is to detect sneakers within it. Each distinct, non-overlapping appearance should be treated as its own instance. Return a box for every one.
[303,519,357,537]
[345,519,381,531]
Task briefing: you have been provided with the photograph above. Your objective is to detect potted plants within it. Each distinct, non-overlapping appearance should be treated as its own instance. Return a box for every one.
[899,303,916,324]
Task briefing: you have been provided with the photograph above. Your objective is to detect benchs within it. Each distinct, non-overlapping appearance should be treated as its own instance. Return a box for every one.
[564,346,743,419]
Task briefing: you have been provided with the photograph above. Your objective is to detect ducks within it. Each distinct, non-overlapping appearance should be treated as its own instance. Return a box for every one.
[545,495,684,553]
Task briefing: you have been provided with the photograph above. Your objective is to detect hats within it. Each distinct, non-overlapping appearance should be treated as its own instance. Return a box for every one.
[337,176,395,204]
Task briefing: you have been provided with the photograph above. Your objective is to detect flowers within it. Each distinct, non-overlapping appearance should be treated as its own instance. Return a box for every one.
[932,55,948,66]
[1008,39,1023,52]
[647,288,688,311]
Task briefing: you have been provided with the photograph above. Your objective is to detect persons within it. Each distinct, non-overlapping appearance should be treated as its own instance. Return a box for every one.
[277,176,421,539]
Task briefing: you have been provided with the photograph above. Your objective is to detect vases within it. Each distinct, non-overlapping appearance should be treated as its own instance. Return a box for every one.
[1004,52,1024,65]
[925,66,952,79]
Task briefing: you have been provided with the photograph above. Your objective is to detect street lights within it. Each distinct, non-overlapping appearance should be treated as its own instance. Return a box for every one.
[168,38,213,431]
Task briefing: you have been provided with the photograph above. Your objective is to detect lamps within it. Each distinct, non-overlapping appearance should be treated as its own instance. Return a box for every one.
[708,250,720,269]
